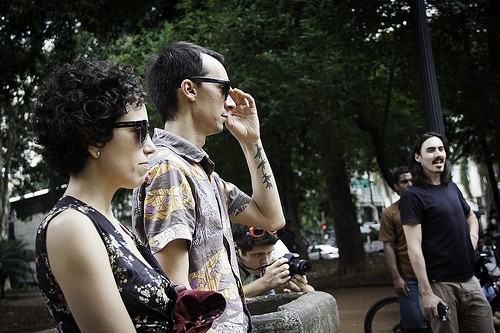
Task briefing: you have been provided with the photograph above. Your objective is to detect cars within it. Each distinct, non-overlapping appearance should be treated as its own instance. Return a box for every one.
[307,244,339,261]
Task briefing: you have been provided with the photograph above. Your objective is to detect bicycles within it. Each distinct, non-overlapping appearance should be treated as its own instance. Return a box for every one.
[362,245,499,333]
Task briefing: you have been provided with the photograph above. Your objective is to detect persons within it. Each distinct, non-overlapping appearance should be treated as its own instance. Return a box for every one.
[232,222,315,298]
[32,57,180,333]
[379,165,435,333]
[399,132,497,333]
[131,41,286,333]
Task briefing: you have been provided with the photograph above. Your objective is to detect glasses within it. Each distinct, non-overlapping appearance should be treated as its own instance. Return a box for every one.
[110,120,149,147]
[245,226,277,239]
[177,77,230,101]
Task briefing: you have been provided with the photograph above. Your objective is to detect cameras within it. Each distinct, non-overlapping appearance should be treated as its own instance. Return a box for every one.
[276,254,312,282]
